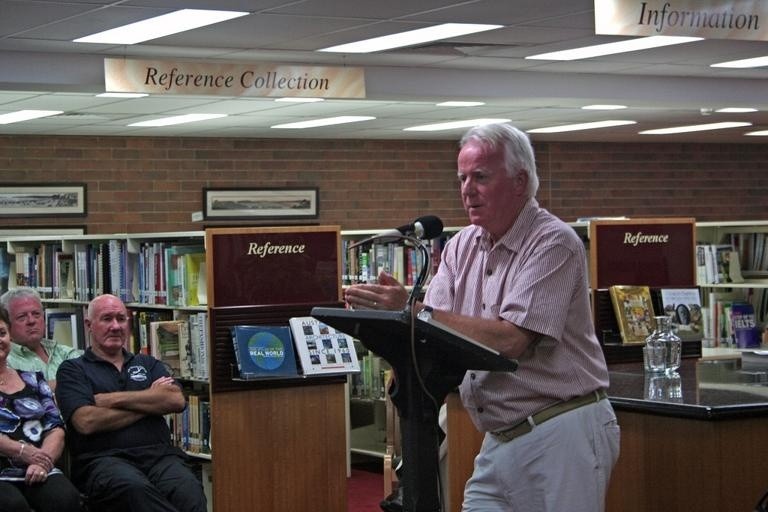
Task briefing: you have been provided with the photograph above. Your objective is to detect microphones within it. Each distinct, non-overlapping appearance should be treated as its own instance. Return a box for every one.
[371,214,448,244]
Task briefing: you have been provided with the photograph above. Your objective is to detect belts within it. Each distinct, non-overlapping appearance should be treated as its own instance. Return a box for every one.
[490,387,609,443]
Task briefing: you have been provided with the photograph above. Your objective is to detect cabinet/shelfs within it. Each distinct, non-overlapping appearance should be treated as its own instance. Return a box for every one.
[339,220,591,496]
[1,220,215,463]
[593,218,766,354]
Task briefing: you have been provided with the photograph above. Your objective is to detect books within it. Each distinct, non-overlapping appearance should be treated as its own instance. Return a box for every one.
[14,237,206,308]
[344,235,459,403]
[689,227,767,353]
[129,311,209,380]
[163,390,209,454]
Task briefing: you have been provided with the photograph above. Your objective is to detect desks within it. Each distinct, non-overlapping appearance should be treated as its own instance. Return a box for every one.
[598,355,766,511]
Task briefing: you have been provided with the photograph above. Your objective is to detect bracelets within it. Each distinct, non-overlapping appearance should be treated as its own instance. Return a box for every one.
[19,444,25,457]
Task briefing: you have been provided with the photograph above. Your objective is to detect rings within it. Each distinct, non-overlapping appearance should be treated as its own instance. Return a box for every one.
[41,471,44,474]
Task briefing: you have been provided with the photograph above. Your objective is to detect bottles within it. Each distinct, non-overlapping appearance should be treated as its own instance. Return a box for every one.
[646,315,681,371]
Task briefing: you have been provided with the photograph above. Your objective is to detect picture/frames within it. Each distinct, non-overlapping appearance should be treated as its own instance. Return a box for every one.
[1,182,88,215]
[204,185,319,219]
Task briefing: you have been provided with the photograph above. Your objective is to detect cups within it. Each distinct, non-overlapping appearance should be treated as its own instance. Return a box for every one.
[642,347,667,373]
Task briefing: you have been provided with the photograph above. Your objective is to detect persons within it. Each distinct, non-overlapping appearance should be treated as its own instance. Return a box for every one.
[336,122,625,509]
[0,306,89,511]
[53,294,210,511]
